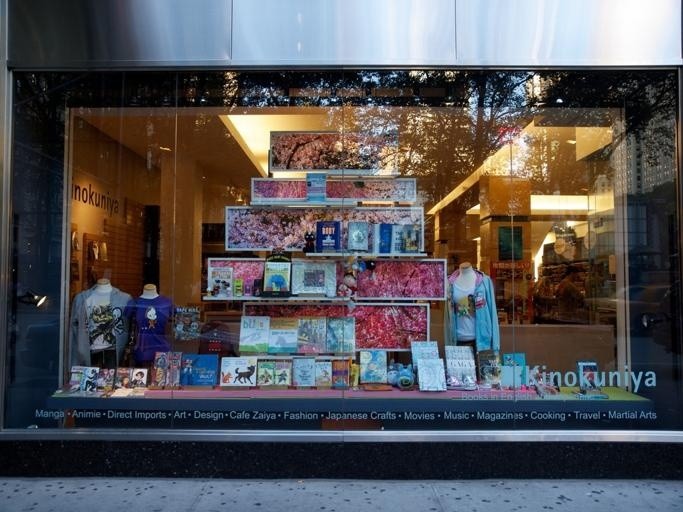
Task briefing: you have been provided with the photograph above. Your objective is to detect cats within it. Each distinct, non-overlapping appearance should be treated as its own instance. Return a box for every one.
[221,371,233,384]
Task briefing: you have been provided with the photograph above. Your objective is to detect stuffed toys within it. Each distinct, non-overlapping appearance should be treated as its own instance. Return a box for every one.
[397,363,415,390]
[387,365,398,386]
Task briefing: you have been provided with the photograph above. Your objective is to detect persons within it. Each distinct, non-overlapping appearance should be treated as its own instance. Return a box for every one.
[131,372,145,388]
[82,369,97,393]
[68,278,133,368]
[447,262,500,380]
[122,284,174,368]
[557,266,584,323]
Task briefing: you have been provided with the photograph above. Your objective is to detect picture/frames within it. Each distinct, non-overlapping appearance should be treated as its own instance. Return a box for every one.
[205,129,448,356]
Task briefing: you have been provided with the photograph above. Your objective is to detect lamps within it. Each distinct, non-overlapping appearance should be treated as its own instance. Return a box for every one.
[12,289,46,307]
[640,314,668,328]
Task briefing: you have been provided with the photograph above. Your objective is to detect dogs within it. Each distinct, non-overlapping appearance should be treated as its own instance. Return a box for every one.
[232,364,256,384]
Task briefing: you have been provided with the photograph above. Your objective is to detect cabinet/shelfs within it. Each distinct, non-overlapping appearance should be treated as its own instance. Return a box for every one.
[201,171,428,361]
